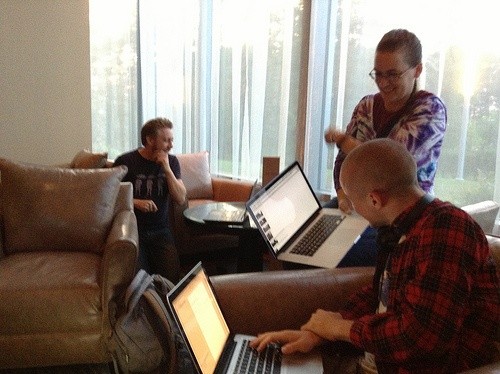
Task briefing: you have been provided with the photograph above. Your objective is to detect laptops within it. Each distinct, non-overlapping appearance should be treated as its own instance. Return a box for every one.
[245,162,369,268]
[165,261,325,374]
[203,180,258,225]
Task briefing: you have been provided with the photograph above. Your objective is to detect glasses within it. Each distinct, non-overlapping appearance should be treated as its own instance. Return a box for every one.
[369,64,416,82]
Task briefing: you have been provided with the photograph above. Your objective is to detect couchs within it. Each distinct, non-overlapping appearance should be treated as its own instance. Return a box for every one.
[105,159,256,214]
[208,234,500,374]
[0,157,140,370]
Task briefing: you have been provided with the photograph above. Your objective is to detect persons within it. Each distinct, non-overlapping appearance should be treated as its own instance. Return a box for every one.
[113,118,186,282]
[283,31,447,269]
[250,141,500,374]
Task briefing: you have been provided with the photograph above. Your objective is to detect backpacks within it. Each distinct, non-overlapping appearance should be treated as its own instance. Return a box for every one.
[108,269,195,374]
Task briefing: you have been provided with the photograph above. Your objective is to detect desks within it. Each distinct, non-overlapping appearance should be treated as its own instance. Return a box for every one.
[182,199,331,271]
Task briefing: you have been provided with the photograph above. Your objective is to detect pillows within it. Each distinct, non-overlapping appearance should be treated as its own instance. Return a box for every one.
[177,148,215,202]
[70,149,108,169]
